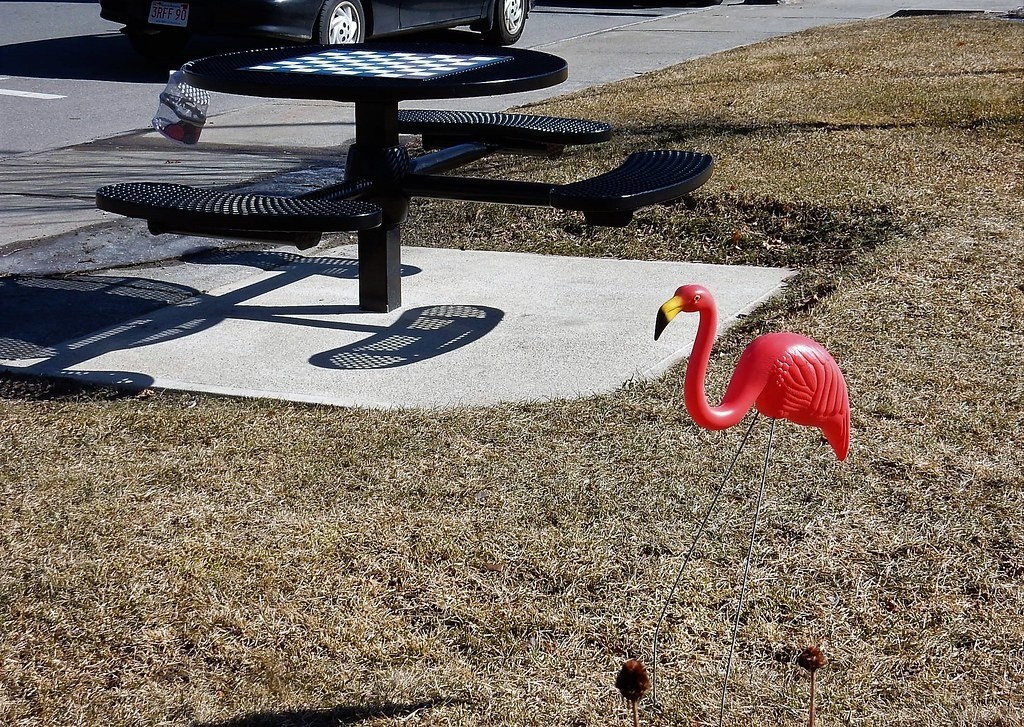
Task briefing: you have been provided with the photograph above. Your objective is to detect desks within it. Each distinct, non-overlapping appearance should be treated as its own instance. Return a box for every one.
[184,41,568,313]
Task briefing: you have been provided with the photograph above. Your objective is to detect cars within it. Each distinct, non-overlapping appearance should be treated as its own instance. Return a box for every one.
[99,1,534,51]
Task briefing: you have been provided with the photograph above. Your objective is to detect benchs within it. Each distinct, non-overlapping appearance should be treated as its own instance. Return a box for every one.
[403,150,715,228]
[397,109,612,178]
[95,178,383,250]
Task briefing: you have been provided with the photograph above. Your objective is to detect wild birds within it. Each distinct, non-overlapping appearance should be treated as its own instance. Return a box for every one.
[612,283,851,727]
